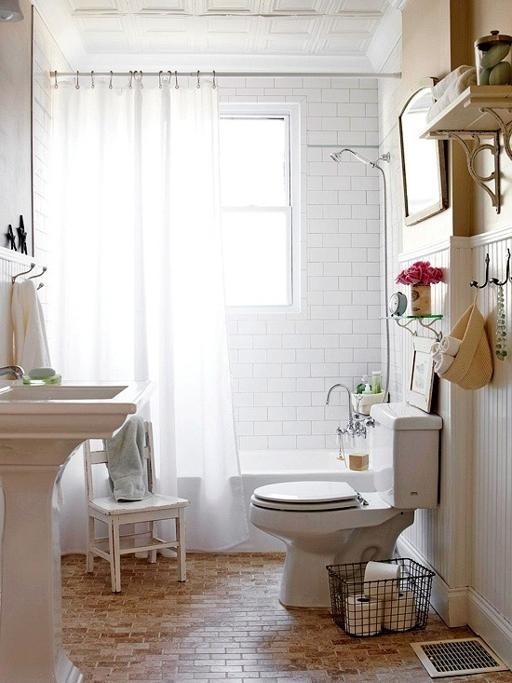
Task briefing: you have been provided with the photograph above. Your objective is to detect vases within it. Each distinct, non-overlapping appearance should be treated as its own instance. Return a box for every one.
[410,284,432,316]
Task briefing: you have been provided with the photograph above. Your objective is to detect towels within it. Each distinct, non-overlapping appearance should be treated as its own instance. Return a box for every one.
[432,343,445,359]
[424,68,476,122]
[431,63,475,100]
[104,414,151,501]
[441,335,461,355]
[434,354,453,374]
[13,279,53,379]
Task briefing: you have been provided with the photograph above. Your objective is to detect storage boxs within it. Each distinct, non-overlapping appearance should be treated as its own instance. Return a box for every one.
[325,558,435,638]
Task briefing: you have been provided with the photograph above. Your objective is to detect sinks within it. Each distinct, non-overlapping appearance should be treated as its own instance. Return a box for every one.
[0,376,154,683]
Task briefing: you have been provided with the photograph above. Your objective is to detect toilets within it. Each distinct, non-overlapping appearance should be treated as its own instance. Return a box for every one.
[249,401,443,608]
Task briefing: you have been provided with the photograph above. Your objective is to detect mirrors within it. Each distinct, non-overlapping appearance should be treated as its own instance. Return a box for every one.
[399,85,448,227]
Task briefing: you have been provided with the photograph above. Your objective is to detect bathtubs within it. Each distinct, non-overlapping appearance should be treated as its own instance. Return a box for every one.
[240,449,374,553]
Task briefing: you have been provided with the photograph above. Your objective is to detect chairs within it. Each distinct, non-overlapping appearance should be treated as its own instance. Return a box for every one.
[84,420,189,594]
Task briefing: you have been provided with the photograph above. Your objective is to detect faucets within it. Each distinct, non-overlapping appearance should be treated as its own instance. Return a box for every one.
[0,364,25,378]
[325,383,367,439]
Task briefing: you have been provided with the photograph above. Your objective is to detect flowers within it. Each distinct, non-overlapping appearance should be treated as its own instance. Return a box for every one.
[394,260,445,316]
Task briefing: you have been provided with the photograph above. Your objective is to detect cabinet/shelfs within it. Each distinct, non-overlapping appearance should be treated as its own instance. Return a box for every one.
[418,84,511,214]
[383,315,441,340]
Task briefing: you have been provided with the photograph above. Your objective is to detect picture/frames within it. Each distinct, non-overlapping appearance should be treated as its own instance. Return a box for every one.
[404,334,440,414]
